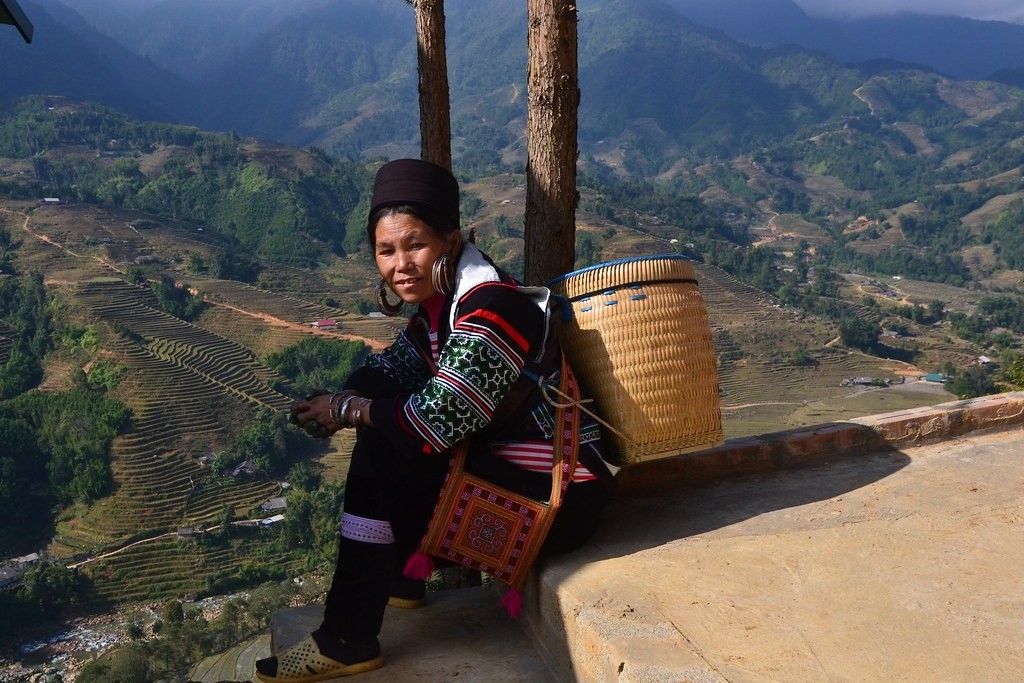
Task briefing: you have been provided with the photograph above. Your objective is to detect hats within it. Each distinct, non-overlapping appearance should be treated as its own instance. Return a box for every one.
[369,159,460,230]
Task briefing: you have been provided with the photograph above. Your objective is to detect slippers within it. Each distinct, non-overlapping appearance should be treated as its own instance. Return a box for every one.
[388,596,425,610]
[256,634,384,683]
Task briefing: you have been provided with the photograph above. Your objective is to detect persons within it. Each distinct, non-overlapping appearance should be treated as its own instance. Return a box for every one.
[255,158,617,683]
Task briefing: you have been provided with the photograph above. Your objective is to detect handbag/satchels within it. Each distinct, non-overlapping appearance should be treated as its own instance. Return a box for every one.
[404,471,558,619]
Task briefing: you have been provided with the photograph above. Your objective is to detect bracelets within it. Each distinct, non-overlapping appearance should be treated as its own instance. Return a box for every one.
[329,394,372,434]
